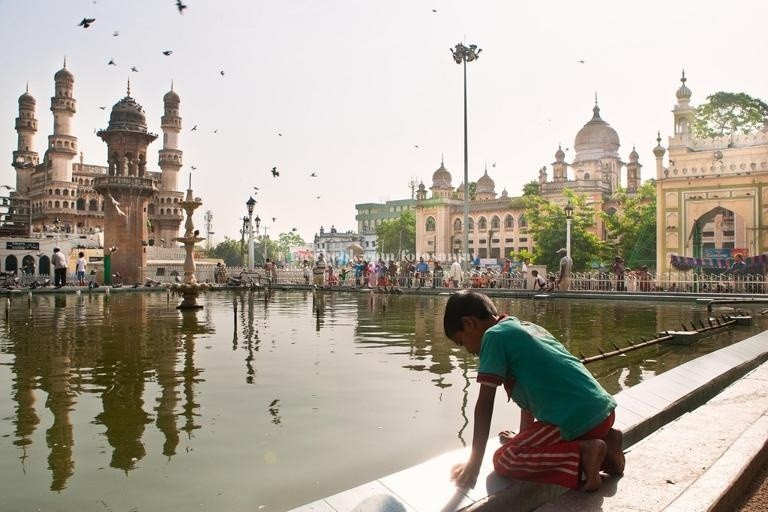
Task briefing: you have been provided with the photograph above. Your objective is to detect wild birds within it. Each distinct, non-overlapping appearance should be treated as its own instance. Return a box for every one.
[107,245,118,255]
[163,50,171,56]
[108,60,117,66]
[1,184,15,191]
[79,16,95,29]
[132,67,138,71]
[271,167,280,179]
[99,106,107,110]
[311,173,319,177]
[191,125,197,131]
[221,70,225,77]
[112,270,121,276]
[177,0,186,13]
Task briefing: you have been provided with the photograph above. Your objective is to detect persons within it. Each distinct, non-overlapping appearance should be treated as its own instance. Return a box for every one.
[264,258,278,283]
[613,255,636,292]
[214,262,227,283]
[313,253,325,284]
[349,253,496,288]
[444,290,627,491]
[531,270,545,291]
[303,260,311,285]
[76,252,87,285]
[636,265,653,292]
[521,257,533,289]
[326,265,333,285]
[339,269,351,285]
[51,246,68,286]
[730,253,748,293]
[500,259,511,286]
[556,248,573,290]
[544,277,555,291]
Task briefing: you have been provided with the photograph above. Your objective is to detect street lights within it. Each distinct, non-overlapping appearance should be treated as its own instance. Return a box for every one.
[53,216,62,248]
[246,196,260,275]
[563,200,573,258]
[450,40,481,287]
[258,216,277,268]
[204,210,213,251]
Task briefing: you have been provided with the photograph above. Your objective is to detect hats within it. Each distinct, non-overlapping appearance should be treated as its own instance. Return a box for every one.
[556,247,567,254]
